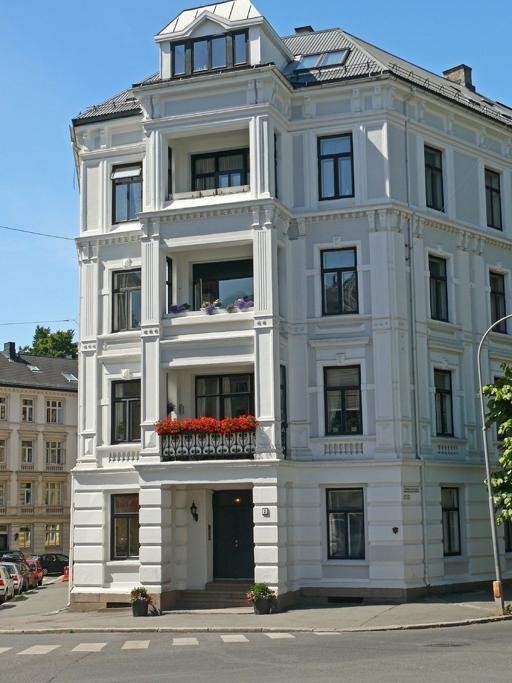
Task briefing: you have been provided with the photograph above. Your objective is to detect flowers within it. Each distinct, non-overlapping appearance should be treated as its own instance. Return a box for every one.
[130,587,151,608]
[153,414,256,435]
[245,583,276,605]
[169,296,254,314]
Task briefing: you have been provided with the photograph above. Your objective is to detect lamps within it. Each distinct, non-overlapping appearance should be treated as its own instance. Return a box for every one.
[190,500,198,522]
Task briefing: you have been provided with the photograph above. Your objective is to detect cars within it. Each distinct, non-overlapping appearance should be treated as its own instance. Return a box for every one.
[0,552,69,602]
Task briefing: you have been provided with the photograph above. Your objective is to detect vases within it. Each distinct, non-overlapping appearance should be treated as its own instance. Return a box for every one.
[254,600,271,615]
[133,600,148,616]
